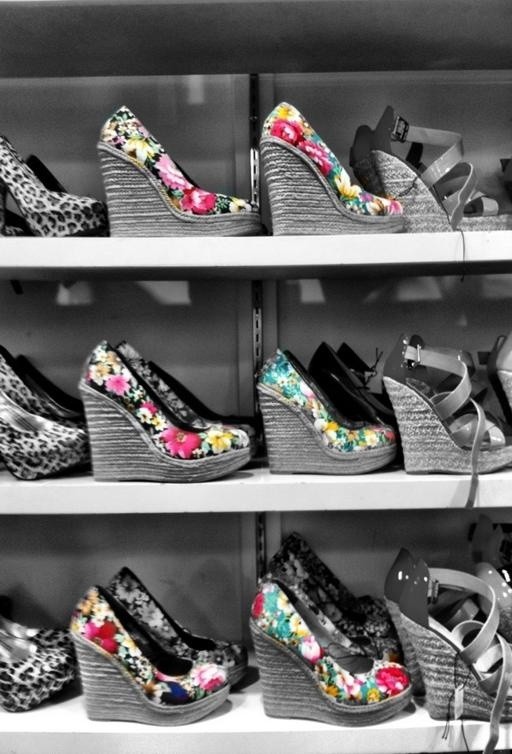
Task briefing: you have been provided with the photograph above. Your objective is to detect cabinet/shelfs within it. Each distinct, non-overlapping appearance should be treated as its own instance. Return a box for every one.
[1,228,511,753]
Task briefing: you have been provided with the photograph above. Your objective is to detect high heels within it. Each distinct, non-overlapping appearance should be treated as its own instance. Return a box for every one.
[0,332,512,483]
[0,533,512,730]
[0,104,512,237]
